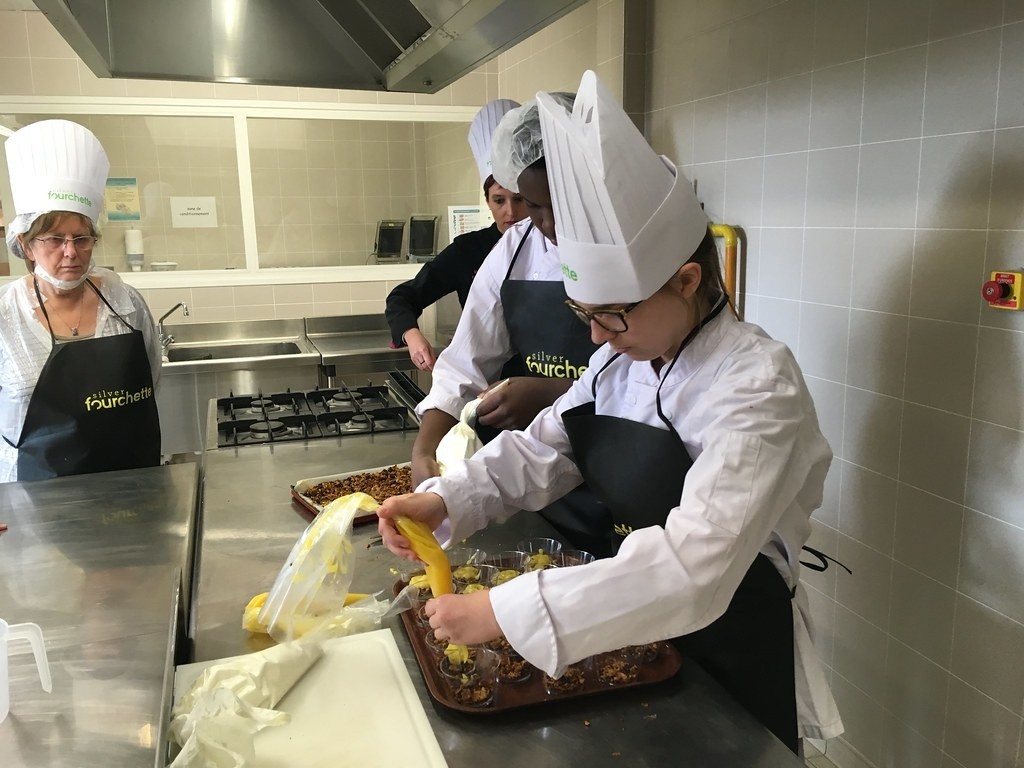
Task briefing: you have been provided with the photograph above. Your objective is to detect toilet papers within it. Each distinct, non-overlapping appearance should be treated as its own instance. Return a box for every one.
[125,230,142,254]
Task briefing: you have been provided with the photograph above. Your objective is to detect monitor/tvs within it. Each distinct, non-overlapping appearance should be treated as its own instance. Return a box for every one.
[406,214,441,259]
[374,220,407,261]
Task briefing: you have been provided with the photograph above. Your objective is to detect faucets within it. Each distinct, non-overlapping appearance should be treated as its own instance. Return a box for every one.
[157,303,189,347]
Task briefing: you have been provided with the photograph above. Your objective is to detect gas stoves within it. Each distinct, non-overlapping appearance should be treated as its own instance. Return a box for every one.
[203,367,428,450]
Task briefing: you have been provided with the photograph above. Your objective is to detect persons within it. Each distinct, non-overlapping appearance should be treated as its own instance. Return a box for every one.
[1,191,163,483]
[409,92,606,494]
[374,200,848,762]
[384,141,534,372]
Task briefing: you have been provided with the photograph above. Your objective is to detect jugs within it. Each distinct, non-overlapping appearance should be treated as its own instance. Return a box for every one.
[0,618,54,724]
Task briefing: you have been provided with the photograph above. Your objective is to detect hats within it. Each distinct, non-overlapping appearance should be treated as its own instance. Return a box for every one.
[4,119,111,224]
[535,70,707,304]
[468,99,522,188]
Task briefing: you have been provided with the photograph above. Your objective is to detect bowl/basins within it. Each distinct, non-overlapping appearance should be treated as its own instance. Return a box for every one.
[593,641,662,688]
[398,538,596,632]
[482,634,534,685]
[537,664,588,698]
[150,261,178,273]
[439,649,502,710]
[423,628,480,678]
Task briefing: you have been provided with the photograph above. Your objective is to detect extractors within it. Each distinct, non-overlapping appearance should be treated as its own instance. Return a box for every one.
[30,0,594,95]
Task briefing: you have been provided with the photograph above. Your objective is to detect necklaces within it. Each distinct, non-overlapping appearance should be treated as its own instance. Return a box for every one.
[35,279,85,336]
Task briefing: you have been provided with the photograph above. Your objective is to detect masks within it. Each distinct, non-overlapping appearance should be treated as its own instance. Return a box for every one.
[34,255,96,290]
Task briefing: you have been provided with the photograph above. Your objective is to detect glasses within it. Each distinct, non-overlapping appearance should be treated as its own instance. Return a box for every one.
[35,236,97,250]
[565,299,643,333]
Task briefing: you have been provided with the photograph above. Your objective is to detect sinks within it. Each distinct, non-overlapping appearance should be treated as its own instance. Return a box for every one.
[163,339,310,364]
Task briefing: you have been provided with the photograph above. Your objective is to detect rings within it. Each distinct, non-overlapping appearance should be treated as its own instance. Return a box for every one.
[421,360,425,365]
